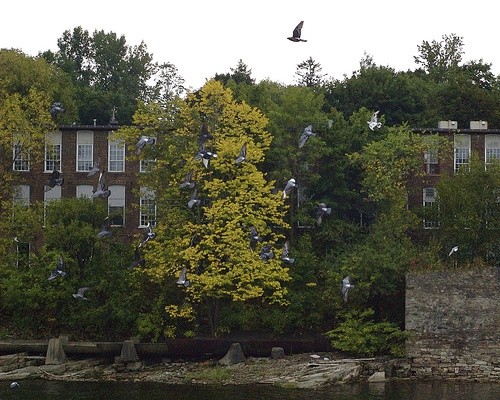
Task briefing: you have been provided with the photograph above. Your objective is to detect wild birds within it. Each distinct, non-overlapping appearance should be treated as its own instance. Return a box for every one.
[2,20,459,394]
